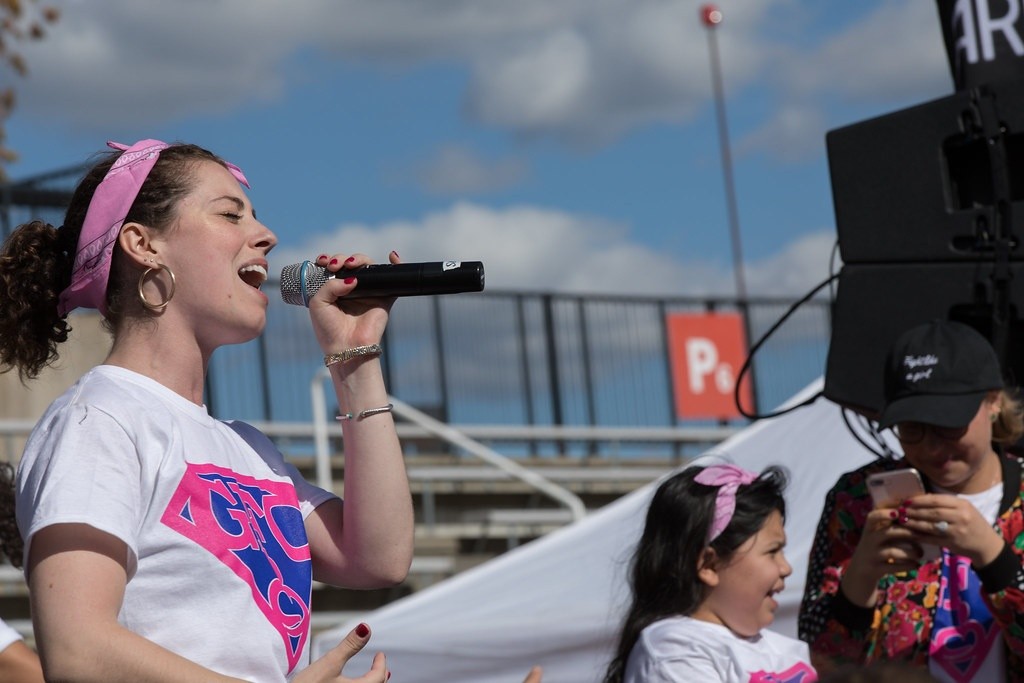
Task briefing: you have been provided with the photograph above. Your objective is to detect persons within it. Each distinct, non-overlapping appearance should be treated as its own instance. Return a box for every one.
[603,456,819,683]
[797,323,1024,683]
[0,138,544,683]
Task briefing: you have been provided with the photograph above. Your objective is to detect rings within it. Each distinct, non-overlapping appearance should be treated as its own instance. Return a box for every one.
[934,520,948,533]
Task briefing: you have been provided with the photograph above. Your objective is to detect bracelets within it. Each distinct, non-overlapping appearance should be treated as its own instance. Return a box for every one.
[324,345,383,367]
[335,404,393,420]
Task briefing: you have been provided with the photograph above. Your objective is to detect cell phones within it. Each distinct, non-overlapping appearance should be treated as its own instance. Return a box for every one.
[867,468,942,563]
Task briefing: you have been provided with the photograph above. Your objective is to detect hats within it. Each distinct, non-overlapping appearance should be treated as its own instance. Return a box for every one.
[877,317,1004,433]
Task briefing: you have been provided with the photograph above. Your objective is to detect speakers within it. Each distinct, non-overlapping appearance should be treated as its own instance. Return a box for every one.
[822,54,1024,421]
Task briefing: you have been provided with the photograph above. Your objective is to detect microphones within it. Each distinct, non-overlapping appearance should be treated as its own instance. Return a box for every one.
[280,260,484,307]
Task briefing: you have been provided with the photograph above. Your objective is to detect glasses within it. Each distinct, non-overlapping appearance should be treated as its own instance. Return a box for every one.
[890,420,968,443]
